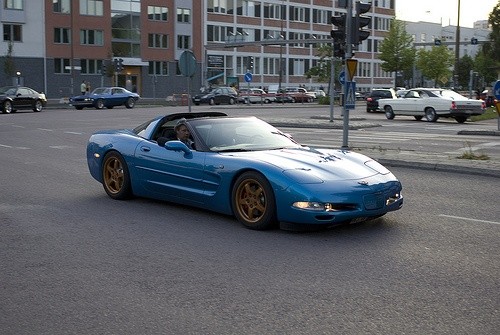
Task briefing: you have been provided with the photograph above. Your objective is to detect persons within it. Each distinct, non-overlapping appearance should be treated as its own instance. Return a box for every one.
[172,117,196,151]
[80,80,92,95]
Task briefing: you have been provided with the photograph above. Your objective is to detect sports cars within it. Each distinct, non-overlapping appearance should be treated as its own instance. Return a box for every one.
[86,111,404,232]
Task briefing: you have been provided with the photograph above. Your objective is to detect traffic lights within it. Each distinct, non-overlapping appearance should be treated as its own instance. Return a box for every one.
[355,0,371,45]
[247,56,254,70]
[119,58,124,72]
[471,38,477,45]
[330,12,347,45]
[114,59,119,72]
[435,40,441,45]
[101,64,106,76]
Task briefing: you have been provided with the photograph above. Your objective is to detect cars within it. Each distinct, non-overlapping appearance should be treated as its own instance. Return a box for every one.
[69,87,140,110]
[192,87,239,106]
[396,90,419,98]
[366,88,398,113]
[237,87,326,105]
[339,92,360,96]
[0,85,48,114]
[378,87,486,123]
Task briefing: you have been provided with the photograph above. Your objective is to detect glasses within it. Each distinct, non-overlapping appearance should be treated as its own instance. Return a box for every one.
[183,129,189,132]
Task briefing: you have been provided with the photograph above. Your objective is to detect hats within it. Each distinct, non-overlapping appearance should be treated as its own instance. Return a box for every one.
[175,118,189,127]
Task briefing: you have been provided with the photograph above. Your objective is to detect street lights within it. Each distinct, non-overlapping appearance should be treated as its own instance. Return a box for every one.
[16,71,21,85]
